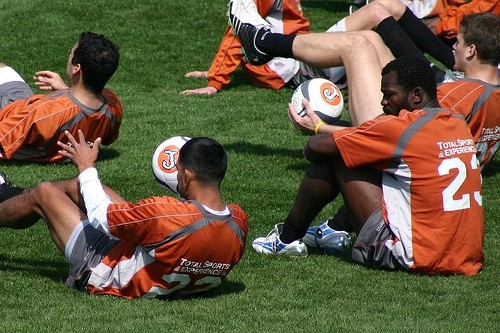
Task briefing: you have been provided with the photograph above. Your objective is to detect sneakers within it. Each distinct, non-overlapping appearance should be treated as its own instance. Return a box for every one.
[226,0,273,65]
[299,217,352,251]
[253,223,308,256]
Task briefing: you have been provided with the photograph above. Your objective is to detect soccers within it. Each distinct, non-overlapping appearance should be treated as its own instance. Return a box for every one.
[291,78,345,125]
[151,135,193,195]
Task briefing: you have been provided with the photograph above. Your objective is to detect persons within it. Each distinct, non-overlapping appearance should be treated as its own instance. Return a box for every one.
[251,55,485,276]
[180,0,500,179]
[0,30,122,164]
[0,130,248,300]
